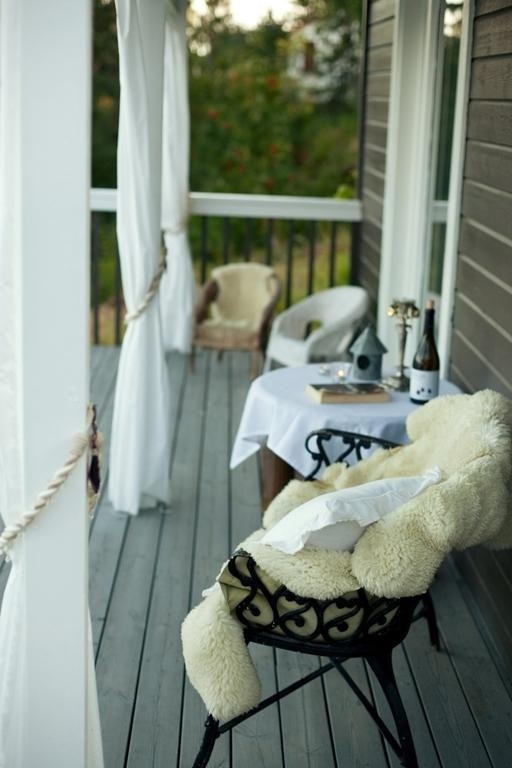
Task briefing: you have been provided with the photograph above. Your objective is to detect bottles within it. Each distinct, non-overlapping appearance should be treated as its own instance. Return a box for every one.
[408,299,441,405]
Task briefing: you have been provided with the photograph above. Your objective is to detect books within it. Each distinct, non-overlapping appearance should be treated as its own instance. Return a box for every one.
[307,381,389,404]
